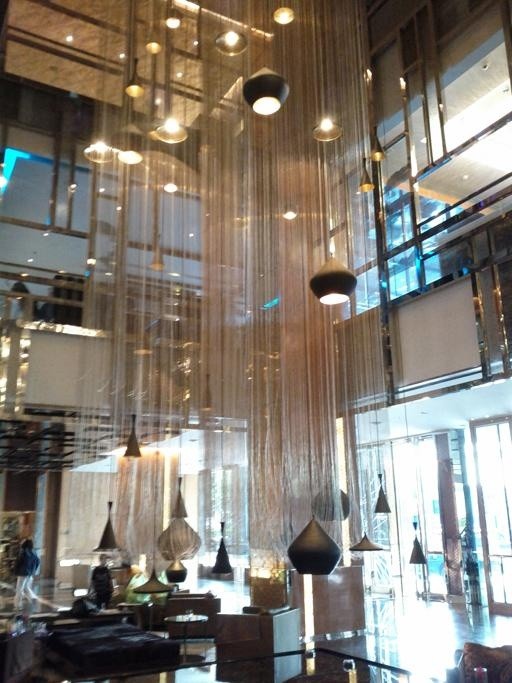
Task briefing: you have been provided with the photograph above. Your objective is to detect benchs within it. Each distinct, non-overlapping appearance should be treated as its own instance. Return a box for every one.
[34,621,186,682]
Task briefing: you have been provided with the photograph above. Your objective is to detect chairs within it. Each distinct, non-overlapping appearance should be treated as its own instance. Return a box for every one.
[164,590,222,638]
[0,618,34,682]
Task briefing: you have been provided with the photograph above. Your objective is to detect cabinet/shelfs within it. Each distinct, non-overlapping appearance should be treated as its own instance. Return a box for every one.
[206,606,305,681]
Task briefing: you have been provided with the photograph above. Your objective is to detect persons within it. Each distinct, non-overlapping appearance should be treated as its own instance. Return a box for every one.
[90,552,115,609]
[11,538,42,615]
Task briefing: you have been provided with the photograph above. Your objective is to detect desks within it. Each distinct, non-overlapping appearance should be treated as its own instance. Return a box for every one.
[115,600,143,629]
[117,620,469,681]
[317,618,510,681]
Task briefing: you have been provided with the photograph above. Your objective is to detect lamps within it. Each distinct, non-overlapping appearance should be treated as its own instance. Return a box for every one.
[59,0,432,595]
